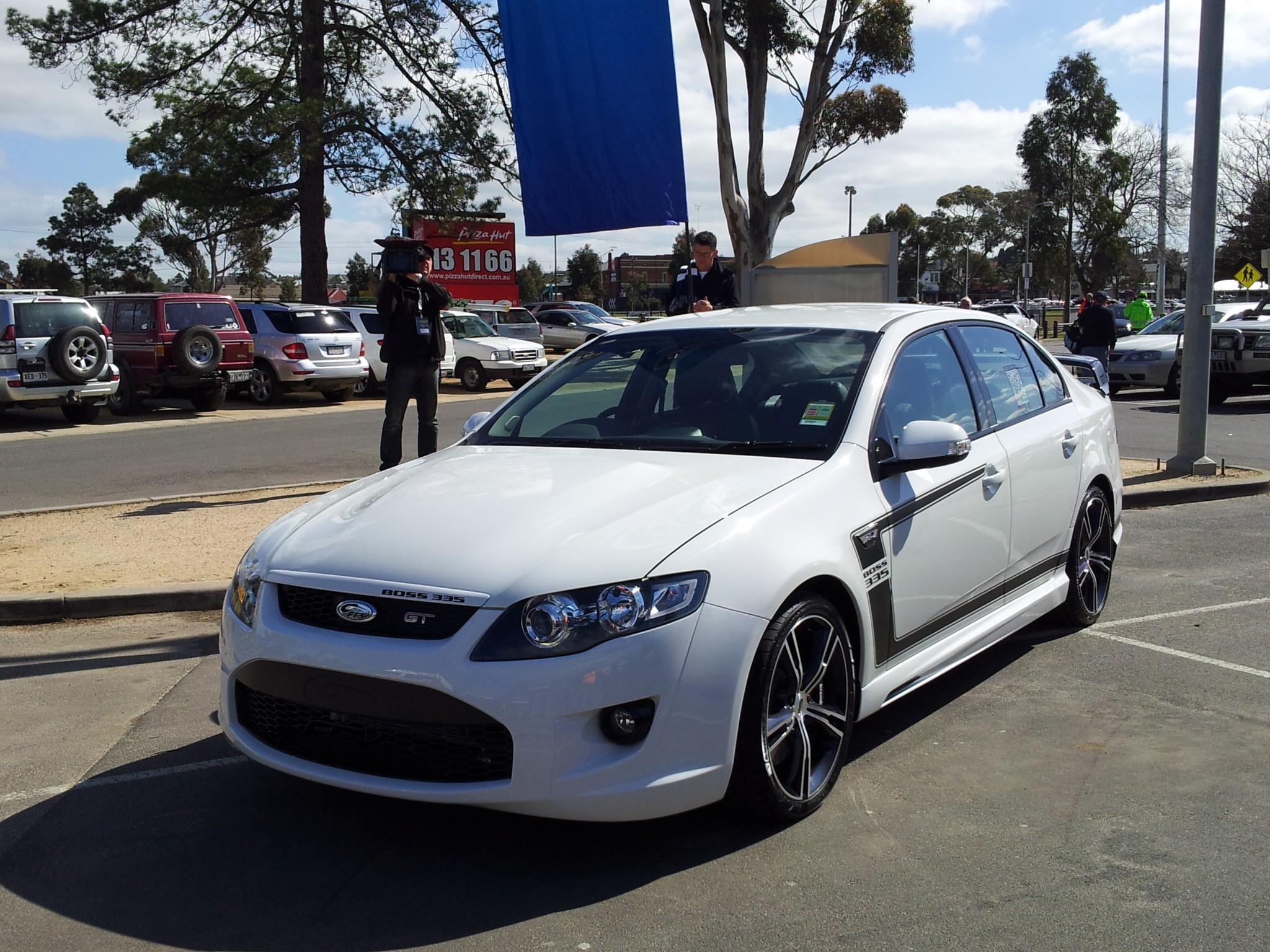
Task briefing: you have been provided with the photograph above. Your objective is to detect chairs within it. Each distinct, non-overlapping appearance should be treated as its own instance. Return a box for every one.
[783,378,848,425]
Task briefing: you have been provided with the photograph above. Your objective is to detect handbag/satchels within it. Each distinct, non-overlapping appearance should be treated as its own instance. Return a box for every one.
[1063,319,1082,353]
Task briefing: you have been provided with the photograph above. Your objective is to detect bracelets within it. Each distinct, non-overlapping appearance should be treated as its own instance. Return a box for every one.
[415,273,422,283]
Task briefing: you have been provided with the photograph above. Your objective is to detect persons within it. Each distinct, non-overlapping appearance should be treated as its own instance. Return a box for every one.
[960,297,972,309]
[907,296,916,303]
[1123,291,1154,331]
[1074,293,1095,317]
[376,236,451,472]
[665,231,740,409]
[1079,291,1116,375]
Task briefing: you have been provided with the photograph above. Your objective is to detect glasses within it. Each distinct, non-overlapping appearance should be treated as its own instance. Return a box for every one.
[694,248,714,257]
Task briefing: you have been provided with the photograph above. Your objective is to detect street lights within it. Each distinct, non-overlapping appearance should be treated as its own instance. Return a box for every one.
[844,185,856,237]
[1023,201,1054,310]
[600,249,614,309]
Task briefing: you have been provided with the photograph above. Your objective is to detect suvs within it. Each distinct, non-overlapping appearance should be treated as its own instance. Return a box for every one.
[75,292,254,417]
[328,303,457,398]
[0,289,121,433]
[448,306,544,346]
[234,300,370,407]
[438,310,547,392]
[523,301,638,353]
[1175,293,1270,409]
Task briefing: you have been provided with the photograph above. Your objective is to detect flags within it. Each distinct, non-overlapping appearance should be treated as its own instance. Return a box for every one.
[498,0,686,237]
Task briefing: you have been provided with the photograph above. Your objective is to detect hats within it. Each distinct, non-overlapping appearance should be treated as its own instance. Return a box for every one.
[1139,291,1148,299]
[1095,291,1108,299]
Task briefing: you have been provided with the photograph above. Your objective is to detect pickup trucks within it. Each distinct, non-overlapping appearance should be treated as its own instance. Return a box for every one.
[977,304,1040,343]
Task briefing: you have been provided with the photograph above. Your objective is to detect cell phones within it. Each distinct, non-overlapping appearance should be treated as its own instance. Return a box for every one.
[696,300,708,306]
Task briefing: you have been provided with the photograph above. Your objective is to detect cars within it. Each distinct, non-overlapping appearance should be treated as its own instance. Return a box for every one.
[209,303,1124,829]
[935,294,1237,339]
[1109,302,1270,399]
[524,309,639,358]
